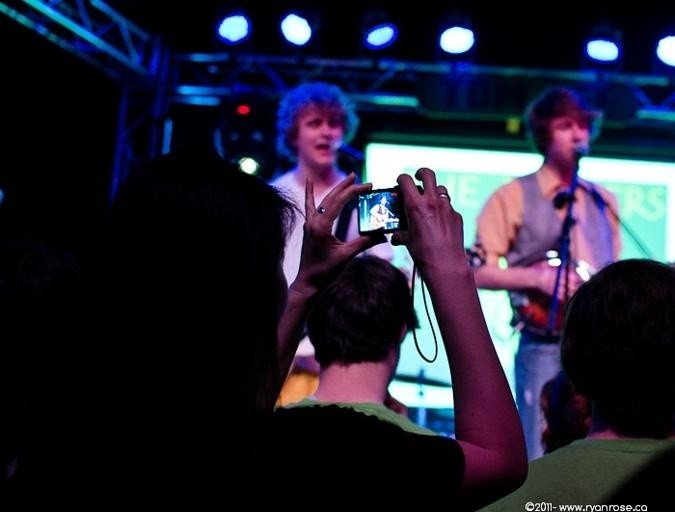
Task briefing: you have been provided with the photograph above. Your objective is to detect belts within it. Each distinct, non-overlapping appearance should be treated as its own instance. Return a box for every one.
[521,329,562,347]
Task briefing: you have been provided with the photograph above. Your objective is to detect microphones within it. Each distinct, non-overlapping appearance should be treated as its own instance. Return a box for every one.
[331,140,364,160]
[569,143,589,156]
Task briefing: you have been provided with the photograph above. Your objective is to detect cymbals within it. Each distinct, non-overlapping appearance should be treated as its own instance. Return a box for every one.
[394,373,449,391]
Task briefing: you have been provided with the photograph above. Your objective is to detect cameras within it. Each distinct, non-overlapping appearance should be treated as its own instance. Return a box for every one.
[357,185,423,237]
